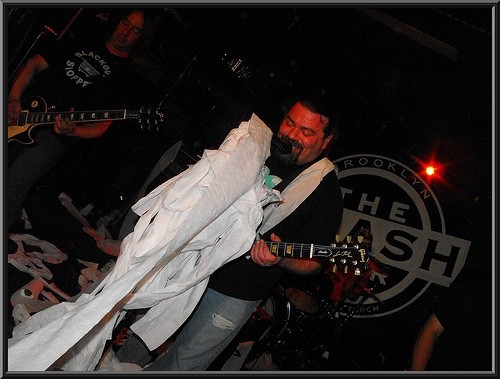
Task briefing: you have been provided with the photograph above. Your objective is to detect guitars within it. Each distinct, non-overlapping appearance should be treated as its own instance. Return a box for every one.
[245,234,369,275]
[8,94,165,145]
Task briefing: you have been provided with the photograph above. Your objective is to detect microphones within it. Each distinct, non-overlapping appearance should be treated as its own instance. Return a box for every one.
[40,21,59,39]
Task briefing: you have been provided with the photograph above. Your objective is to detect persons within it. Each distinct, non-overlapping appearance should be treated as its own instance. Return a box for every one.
[7,3,152,232]
[116,84,342,372]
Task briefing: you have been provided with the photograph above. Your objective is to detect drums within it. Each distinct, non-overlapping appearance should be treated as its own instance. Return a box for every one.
[285,269,334,313]
[241,283,291,339]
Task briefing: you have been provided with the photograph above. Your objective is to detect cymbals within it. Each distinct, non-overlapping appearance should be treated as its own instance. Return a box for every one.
[358,282,384,305]
[367,255,390,274]
